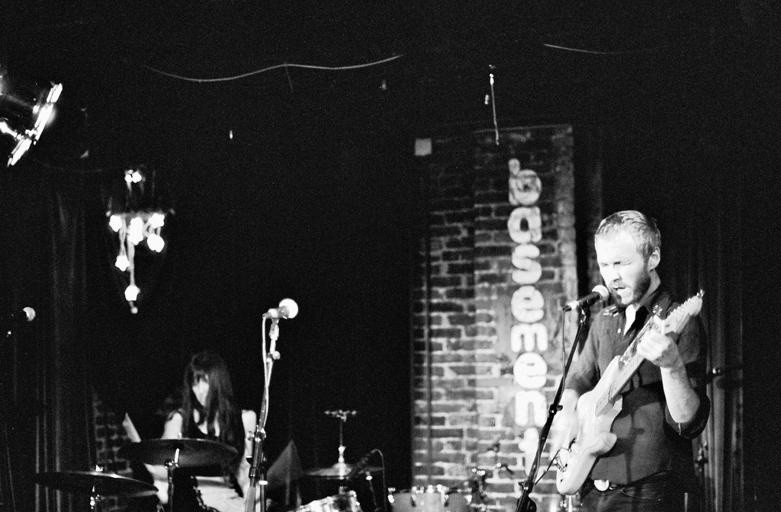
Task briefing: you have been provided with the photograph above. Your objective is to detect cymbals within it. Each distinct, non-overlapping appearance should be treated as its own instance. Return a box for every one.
[32,471,158,495]
[116,439,236,467]
[303,465,384,478]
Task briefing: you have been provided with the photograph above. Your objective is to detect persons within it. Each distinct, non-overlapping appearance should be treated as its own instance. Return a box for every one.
[123,349,260,512]
[550,210,711,511]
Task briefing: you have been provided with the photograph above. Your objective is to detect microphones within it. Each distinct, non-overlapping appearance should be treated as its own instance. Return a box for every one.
[563,285,610,311]
[263,298,299,319]
[0,307,36,329]
[342,450,371,483]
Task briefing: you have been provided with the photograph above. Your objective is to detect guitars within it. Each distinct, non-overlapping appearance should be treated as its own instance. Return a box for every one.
[556,292,703,495]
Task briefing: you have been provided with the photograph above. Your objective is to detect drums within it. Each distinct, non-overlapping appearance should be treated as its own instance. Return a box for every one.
[286,490,364,512]
[388,484,484,511]
